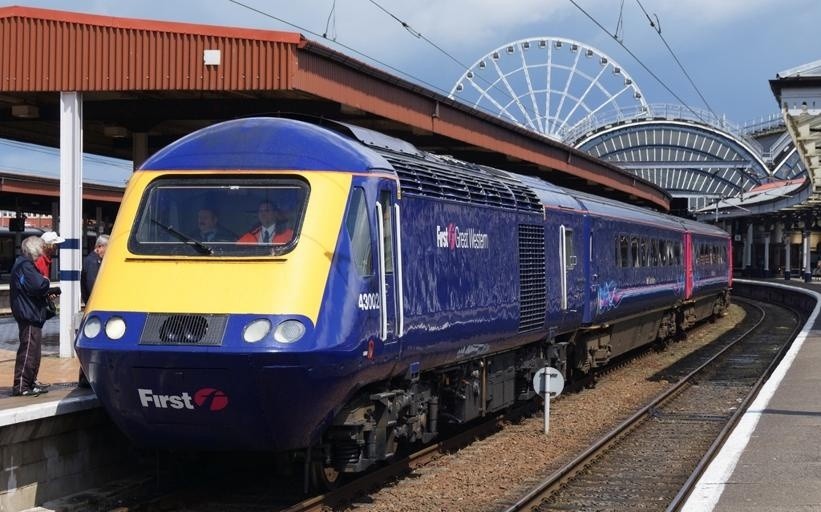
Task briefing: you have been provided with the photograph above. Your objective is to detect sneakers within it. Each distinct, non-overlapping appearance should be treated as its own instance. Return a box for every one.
[14,379,51,397]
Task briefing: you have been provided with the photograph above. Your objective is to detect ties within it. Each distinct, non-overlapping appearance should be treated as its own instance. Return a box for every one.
[262,230,271,243]
[203,232,211,242]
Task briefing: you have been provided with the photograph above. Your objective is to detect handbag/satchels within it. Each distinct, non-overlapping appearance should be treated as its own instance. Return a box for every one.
[44,294,57,320]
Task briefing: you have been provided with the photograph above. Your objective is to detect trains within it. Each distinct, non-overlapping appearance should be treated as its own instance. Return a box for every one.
[72,116,732,503]
[0,224,97,281]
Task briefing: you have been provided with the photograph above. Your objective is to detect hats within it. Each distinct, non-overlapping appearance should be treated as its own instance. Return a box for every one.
[40,231,65,245]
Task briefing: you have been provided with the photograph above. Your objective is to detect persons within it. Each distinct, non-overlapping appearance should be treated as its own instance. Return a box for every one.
[78,235,111,388]
[9,236,56,397]
[190,203,240,241]
[34,230,67,280]
[236,200,294,242]
